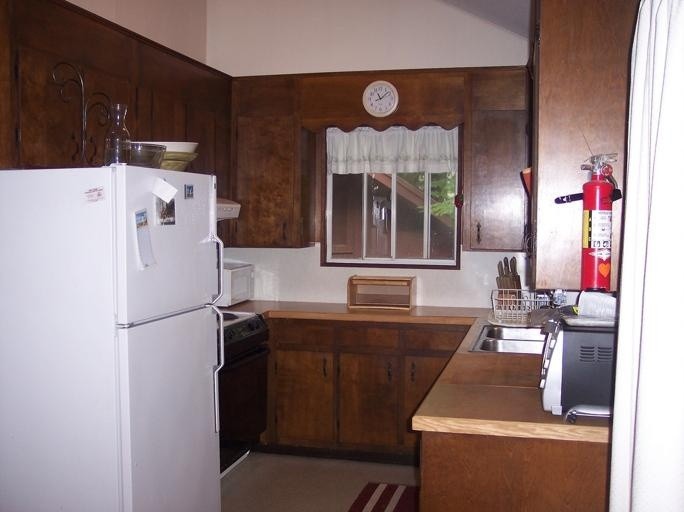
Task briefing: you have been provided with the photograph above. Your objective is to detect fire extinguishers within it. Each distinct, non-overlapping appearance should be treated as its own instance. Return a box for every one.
[581,153,616,292]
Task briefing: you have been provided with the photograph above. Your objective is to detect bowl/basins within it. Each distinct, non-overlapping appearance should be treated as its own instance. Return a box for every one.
[128,141,199,170]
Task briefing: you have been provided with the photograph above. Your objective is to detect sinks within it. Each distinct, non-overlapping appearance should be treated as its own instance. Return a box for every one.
[467,338,546,354]
[476,323,548,342]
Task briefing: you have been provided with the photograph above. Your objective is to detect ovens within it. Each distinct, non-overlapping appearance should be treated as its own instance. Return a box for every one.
[217,314,268,473]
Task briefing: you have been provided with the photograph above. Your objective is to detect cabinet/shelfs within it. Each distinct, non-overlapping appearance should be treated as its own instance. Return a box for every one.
[336,321,402,463]
[404,323,473,464]
[229,72,314,252]
[528,2,637,289]
[2,2,231,247]
[266,324,338,457]
[418,434,611,512]
[460,66,534,254]
[219,332,269,479]
[315,68,465,133]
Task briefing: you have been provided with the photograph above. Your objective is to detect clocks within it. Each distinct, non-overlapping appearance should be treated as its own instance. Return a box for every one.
[362,80,400,118]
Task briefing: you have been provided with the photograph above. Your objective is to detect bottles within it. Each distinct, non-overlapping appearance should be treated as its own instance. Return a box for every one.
[104,103,131,166]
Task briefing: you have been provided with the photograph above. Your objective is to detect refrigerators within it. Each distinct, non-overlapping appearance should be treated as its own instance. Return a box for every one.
[1,161,226,510]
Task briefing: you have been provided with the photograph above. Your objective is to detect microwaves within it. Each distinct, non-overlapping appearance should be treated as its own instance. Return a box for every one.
[215,263,255,308]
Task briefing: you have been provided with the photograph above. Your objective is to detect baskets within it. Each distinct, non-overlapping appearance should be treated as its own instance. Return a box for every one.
[492,288,554,324]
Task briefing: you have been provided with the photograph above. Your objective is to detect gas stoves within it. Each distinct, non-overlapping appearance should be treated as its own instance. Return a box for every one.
[217,311,256,329]
